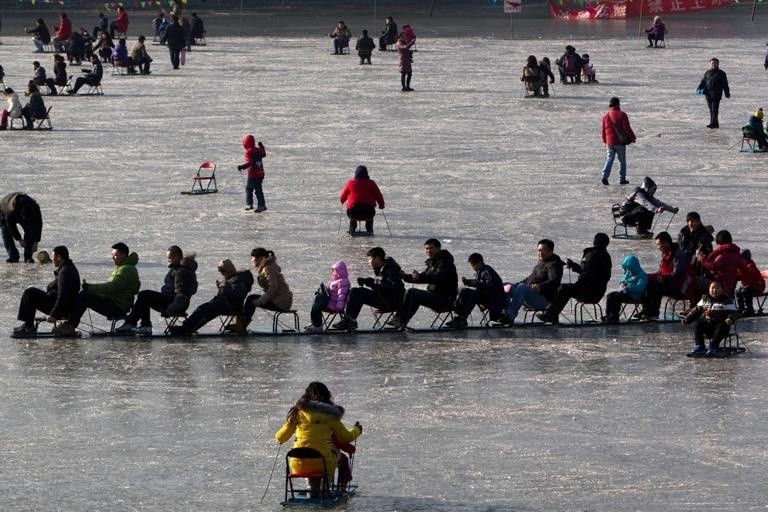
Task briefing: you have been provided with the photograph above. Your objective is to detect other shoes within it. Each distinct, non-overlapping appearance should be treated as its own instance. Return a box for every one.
[498,313,512,324]
[634,311,659,319]
[602,313,619,323]
[446,317,468,328]
[305,325,324,332]
[55,323,75,334]
[390,320,402,327]
[680,310,690,317]
[13,324,35,336]
[743,310,754,316]
[131,326,153,335]
[115,324,135,333]
[172,326,190,336]
[228,324,246,333]
[332,318,357,329]
[536,313,557,324]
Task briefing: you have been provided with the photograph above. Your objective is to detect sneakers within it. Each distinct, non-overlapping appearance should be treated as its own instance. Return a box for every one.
[254,205,268,213]
[244,204,254,211]
[5,257,20,263]
[619,180,631,185]
[24,258,35,263]
[601,178,609,186]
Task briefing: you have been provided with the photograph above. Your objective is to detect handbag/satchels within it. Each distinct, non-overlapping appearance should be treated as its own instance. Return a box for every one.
[615,126,632,145]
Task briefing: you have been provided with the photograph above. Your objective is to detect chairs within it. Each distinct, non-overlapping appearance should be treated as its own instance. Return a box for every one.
[740,126,768,152]
[347,202,375,237]
[656,34,665,48]
[0,32,206,129]
[612,203,653,238]
[340,32,417,54]
[280,448,358,507]
[191,161,217,191]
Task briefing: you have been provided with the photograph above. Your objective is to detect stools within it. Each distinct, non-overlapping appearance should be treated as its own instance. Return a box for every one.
[524,73,598,97]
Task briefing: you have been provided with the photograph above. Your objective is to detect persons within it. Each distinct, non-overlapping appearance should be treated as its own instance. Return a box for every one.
[274,379,363,498]
[696,58,731,130]
[0,191,43,265]
[330,16,417,93]
[599,97,637,186]
[237,135,270,213]
[679,278,741,360]
[520,45,600,100]
[0,1,205,135]
[742,107,768,148]
[12,245,292,335]
[339,166,386,238]
[644,14,666,49]
[613,177,682,240]
[306,212,765,334]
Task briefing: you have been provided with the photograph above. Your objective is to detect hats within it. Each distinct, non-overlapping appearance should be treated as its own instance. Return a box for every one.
[755,107,763,119]
